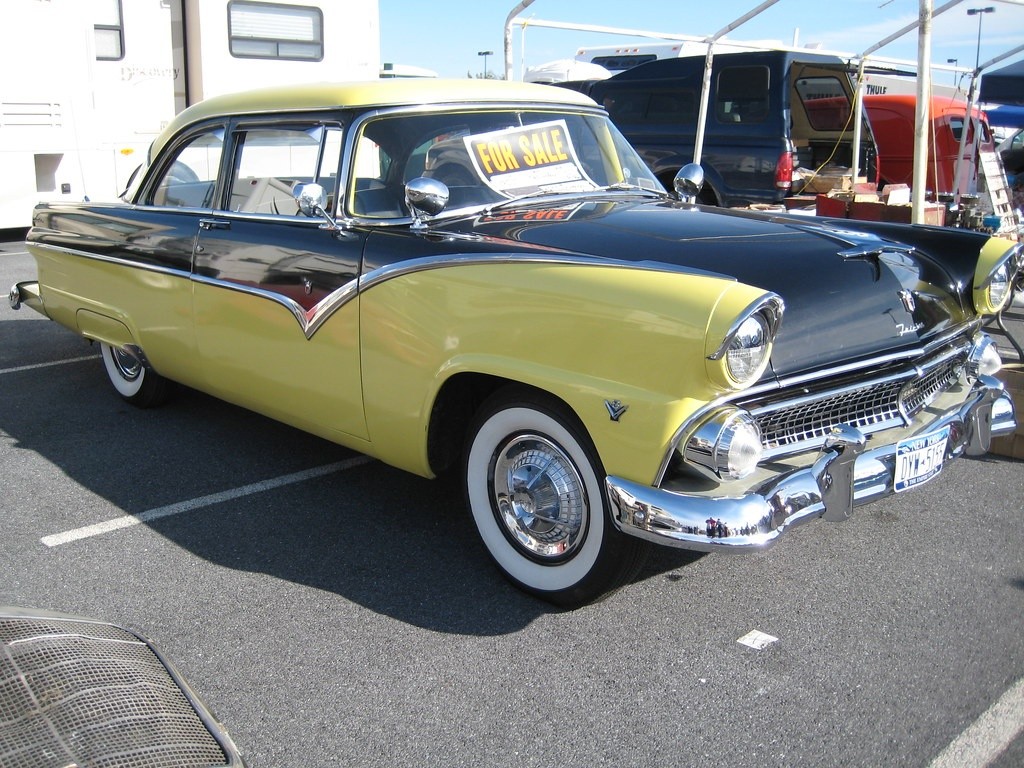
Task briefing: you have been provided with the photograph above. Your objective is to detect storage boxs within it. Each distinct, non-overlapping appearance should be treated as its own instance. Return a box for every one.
[805,177,849,193]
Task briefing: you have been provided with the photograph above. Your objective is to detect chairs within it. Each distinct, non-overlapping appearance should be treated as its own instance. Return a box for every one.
[324,193,365,217]
[355,186,404,217]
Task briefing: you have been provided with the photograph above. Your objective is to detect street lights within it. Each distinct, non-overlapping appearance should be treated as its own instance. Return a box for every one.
[968,5,995,69]
[948,59,958,85]
[477,51,494,76]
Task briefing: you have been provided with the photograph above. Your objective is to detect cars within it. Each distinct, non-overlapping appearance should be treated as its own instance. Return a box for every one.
[10,76,1023,610]
[378,50,885,218]
[804,94,1024,244]
